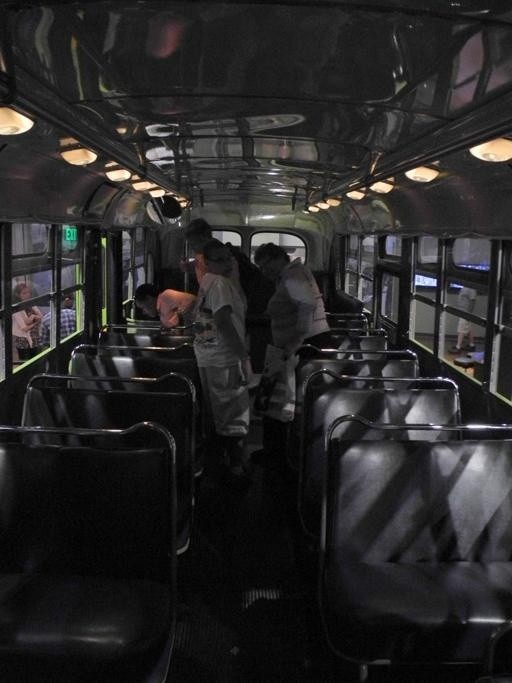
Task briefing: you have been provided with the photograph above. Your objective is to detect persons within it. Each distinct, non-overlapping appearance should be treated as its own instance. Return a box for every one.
[137,283,201,336]
[177,215,260,372]
[10,281,45,363]
[251,241,334,426]
[37,287,77,349]
[195,238,262,493]
[446,281,478,353]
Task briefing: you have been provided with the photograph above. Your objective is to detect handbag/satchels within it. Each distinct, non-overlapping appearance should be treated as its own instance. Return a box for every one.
[252,343,300,423]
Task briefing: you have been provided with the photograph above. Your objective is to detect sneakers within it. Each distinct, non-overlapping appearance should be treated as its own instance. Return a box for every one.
[213,447,287,491]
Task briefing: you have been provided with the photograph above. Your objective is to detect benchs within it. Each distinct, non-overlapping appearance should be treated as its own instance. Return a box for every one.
[293,269,512,683]
[0,308,201,683]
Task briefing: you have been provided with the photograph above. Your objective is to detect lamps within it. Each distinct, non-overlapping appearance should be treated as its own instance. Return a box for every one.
[302,135,512,214]
[1,101,192,226]
